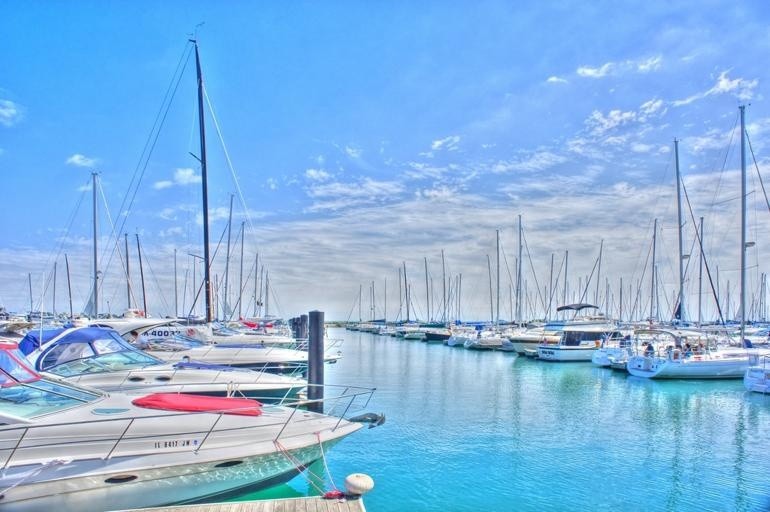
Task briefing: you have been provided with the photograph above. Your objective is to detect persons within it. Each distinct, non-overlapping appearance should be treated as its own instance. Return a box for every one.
[543,338,548,346]
[593,338,600,347]
[672,343,703,360]
[644,343,654,357]
